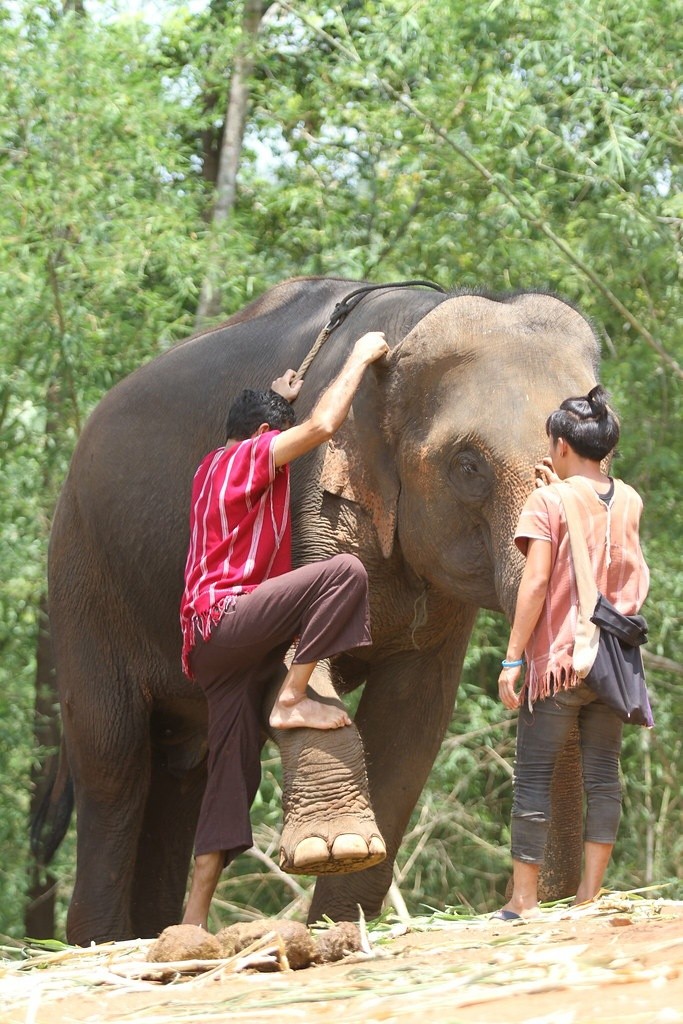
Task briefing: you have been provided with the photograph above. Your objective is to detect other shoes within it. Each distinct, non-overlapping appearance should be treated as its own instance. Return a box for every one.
[489,908,518,922]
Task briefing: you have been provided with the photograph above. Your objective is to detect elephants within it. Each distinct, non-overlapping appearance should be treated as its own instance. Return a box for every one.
[30,275,601,950]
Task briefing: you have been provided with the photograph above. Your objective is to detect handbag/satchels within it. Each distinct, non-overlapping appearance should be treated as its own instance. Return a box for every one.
[581,593,655,726]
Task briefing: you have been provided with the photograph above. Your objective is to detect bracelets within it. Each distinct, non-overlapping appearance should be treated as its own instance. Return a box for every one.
[501,660,524,669]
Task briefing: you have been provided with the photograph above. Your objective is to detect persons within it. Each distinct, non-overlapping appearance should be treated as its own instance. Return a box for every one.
[178,331,389,935]
[483,385,654,921]
